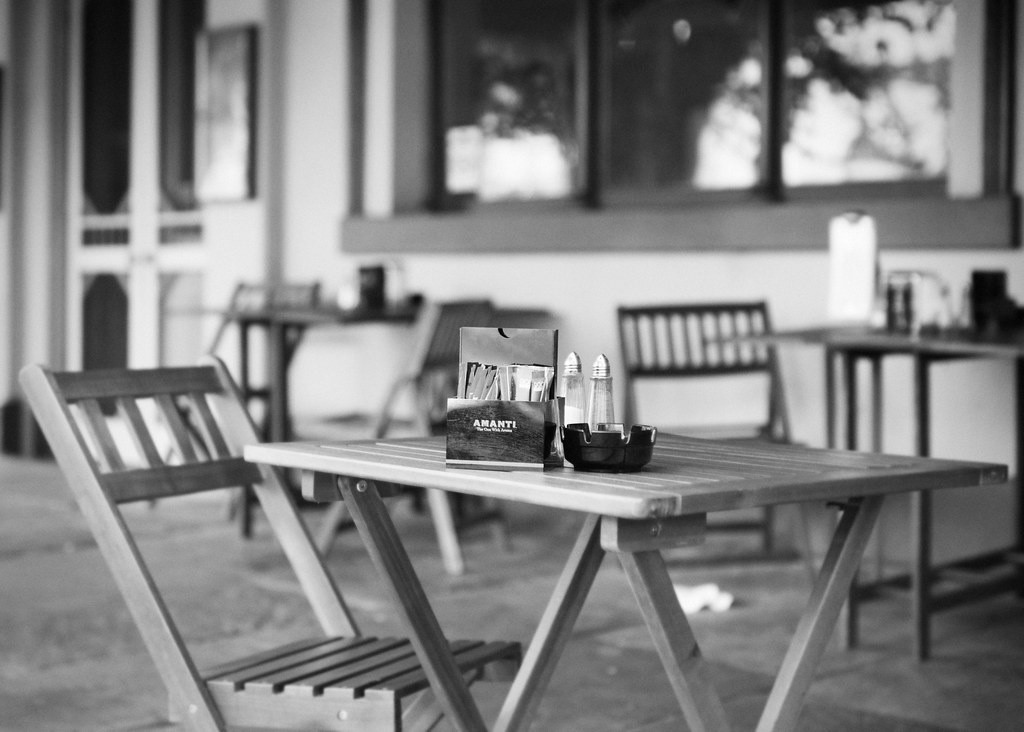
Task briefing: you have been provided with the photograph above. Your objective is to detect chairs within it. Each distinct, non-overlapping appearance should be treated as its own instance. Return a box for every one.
[616,299,792,558]
[145,279,320,520]
[18,355,523,732]
[315,300,511,574]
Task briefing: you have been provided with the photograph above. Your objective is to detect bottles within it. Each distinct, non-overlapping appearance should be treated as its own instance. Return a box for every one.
[587,354,615,436]
[559,351,586,428]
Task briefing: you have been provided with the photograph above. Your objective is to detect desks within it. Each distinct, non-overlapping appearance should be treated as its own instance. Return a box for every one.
[242,433,1006,732]
[224,302,548,550]
[708,327,1024,664]
